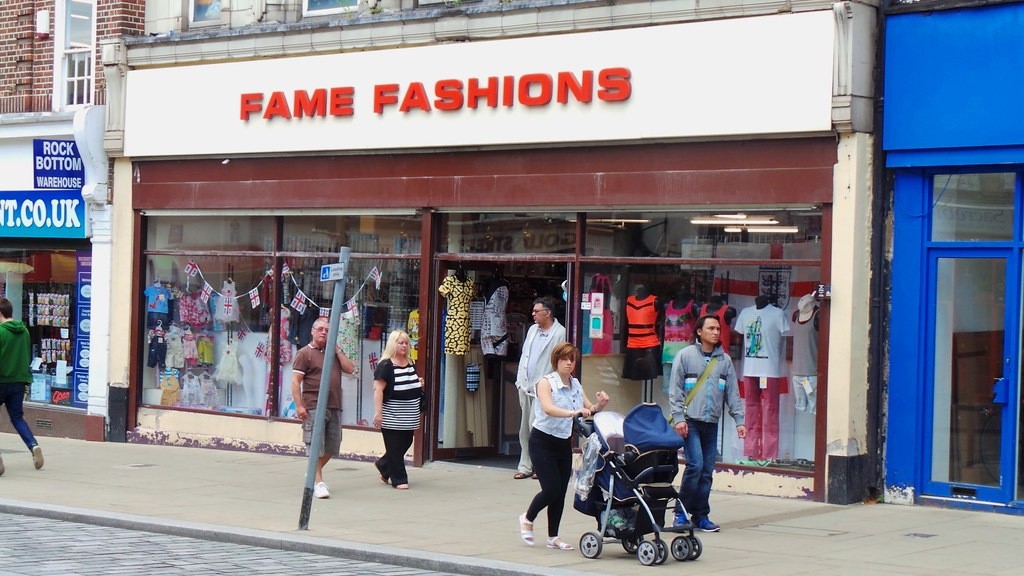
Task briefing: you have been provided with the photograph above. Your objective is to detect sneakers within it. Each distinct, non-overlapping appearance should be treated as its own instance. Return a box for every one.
[313,480,330,498]
[672,513,689,533]
[0,453,5,476]
[29,442,44,469]
[691,516,720,532]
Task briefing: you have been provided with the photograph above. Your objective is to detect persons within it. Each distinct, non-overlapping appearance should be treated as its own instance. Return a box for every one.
[621,282,665,379]
[290,317,354,499]
[513,295,568,480]
[518,341,609,550]
[658,282,698,396]
[586,270,615,355]
[0,297,45,478]
[789,292,823,414]
[439,267,478,355]
[478,277,512,361]
[668,313,747,534]
[373,328,425,490]
[734,292,791,461]
[694,292,738,361]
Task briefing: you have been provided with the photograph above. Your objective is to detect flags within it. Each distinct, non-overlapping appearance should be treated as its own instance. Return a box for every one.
[184,253,384,361]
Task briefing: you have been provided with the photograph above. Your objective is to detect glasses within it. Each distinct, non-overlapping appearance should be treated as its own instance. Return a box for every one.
[312,326,328,333]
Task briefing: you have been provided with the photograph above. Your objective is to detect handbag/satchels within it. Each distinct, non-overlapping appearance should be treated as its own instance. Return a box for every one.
[667,405,687,429]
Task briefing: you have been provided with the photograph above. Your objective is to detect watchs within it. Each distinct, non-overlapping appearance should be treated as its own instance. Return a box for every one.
[336,347,344,353]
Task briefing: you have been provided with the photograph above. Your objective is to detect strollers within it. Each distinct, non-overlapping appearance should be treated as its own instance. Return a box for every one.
[571,403,704,565]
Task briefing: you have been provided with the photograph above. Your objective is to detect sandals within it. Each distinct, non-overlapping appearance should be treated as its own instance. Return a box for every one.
[519,512,534,546]
[547,537,574,550]
[514,470,538,479]
[374,461,409,489]
